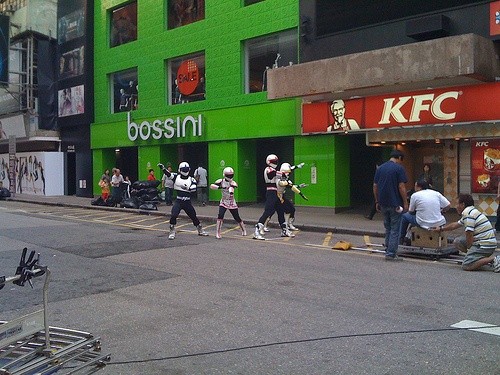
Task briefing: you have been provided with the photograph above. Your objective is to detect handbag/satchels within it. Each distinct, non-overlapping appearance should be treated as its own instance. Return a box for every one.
[106,198,115,207]
[194,170,200,182]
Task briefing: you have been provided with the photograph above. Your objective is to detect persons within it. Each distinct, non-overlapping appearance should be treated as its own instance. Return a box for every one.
[366,162,381,220]
[148,169,156,181]
[174,80,190,103]
[495,178,500,233]
[61,88,77,116]
[253,154,309,240]
[194,162,207,206]
[60,46,84,77]
[99,168,131,207]
[119,80,138,111]
[162,165,174,205]
[373,149,408,262]
[158,162,209,239]
[428,194,500,273]
[210,167,247,239]
[398,177,450,244]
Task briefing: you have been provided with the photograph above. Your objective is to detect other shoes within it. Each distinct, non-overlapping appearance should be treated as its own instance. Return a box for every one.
[199,231,209,236]
[490,255,500,272]
[384,255,403,261]
[168,232,176,239]
[365,215,372,220]
[166,202,173,206]
[203,203,206,206]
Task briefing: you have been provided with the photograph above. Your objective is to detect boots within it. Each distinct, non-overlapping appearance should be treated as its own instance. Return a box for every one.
[216,219,223,239]
[238,222,246,235]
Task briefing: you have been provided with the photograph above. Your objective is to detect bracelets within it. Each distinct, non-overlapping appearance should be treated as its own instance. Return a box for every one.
[376,202,378,203]
[440,226,443,231]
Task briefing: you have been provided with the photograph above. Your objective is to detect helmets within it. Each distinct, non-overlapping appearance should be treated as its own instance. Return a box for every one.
[222,167,235,182]
[266,154,278,168]
[178,162,190,176]
[280,162,291,173]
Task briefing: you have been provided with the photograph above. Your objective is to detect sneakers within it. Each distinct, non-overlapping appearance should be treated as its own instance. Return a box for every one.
[286,224,299,230]
[281,230,295,237]
[253,234,265,240]
